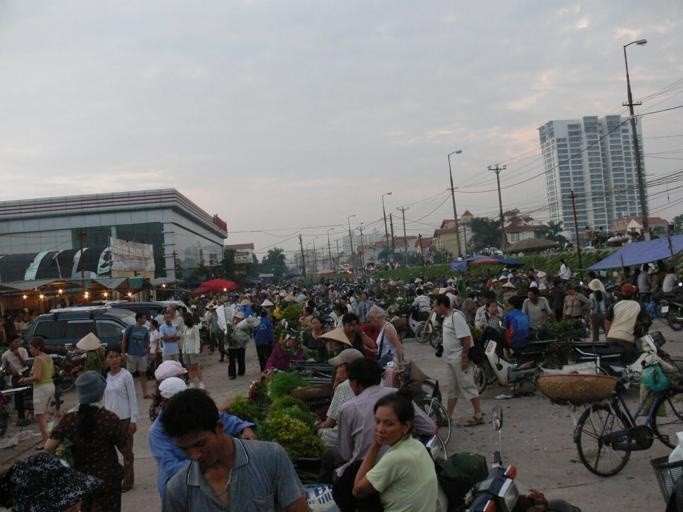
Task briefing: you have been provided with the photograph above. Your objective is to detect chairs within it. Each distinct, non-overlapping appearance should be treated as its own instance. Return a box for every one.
[97,327,108,338]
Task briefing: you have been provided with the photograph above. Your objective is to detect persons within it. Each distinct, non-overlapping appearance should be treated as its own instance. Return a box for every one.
[1,227,682,511]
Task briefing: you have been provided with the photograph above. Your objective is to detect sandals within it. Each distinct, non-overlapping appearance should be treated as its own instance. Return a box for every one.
[434,415,486,428]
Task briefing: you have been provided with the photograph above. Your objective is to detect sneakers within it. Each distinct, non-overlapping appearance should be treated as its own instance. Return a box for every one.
[189,381,208,391]
[493,393,513,401]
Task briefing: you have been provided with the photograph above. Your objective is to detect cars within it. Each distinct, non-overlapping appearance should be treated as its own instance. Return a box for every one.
[484,247,504,258]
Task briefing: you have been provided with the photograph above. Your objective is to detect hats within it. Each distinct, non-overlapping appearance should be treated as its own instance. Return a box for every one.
[158,376,188,400]
[316,327,353,347]
[327,348,365,367]
[75,370,107,404]
[233,294,294,319]
[587,279,605,291]
[621,283,637,297]
[76,332,102,351]
[0,451,107,512]
[153,359,188,382]
[490,269,548,291]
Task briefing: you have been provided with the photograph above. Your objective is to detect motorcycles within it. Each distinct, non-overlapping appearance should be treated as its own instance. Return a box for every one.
[566,312,667,388]
[40,342,111,392]
[399,306,446,349]
[654,287,683,333]
[461,329,554,397]
[462,402,547,510]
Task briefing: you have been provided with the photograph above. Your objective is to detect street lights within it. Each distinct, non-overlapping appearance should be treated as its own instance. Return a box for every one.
[623,38,647,239]
[381,191,396,266]
[446,149,466,264]
[298,228,336,274]
[347,214,360,257]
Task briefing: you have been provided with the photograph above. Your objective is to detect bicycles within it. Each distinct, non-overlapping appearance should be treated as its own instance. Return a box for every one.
[403,375,453,464]
[571,365,682,476]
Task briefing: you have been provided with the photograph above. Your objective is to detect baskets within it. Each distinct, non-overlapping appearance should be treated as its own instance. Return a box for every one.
[536,371,618,402]
[289,381,334,402]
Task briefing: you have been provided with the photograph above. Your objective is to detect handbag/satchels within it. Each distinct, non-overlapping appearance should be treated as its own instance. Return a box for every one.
[637,382,667,418]
[377,349,427,387]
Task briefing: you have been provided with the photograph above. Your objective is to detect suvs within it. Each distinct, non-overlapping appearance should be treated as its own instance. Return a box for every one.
[103,299,190,323]
[24,304,140,361]
